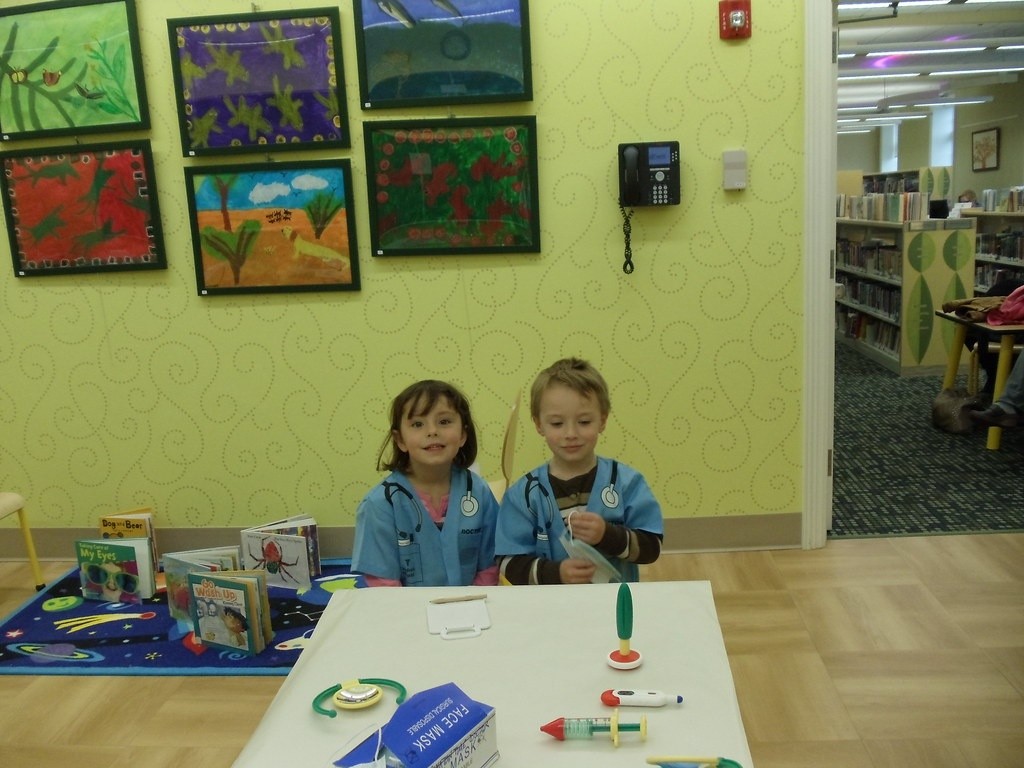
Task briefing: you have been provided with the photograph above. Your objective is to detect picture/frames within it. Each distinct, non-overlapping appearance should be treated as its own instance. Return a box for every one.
[970,127,1001,173]
[354,1,534,110]
[0,1,151,142]
[363,117,545,257]
[165,7,353,158]
[184,159,363,297]
[0,140,169,279]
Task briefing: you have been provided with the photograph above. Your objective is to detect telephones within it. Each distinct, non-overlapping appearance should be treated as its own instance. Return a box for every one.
[618,140,681,207]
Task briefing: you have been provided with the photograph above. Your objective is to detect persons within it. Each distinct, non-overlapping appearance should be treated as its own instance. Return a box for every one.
[350,377,504,586]
[970,346,1024,429]
[958,190,981,207]
[494,359,661,585]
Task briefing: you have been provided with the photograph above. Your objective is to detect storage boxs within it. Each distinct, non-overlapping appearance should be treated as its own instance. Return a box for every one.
[333,682,499,768]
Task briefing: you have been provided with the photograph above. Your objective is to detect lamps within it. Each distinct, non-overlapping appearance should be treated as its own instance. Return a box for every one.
[837,0,1024,135]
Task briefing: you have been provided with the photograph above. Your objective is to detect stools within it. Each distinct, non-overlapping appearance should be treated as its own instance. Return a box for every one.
[0,494,46,592]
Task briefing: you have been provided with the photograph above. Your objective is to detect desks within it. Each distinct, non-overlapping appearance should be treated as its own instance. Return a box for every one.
[936,308,1024,450]
[230,580,755,768]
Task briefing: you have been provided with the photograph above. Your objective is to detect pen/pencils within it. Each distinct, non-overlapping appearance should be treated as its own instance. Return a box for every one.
[429,594,488,604]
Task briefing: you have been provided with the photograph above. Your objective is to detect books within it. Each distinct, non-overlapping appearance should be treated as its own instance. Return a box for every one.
[187,570,272,655]
[73,536,157,604]
[837,192,928,223]
[975,265,1024,290]
[161,545,242,622]
[101,507,159,572]
[976,231,1024,262]
[984,185,1023,212]
[240,514,321,589]
[836,238,901,359]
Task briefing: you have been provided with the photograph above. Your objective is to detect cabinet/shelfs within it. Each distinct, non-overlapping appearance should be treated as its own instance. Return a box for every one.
[834,213,979,375]
[962,211,1024,297]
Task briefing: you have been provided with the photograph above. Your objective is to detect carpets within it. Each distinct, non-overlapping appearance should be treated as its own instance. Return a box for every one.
[0,560,356,674]
